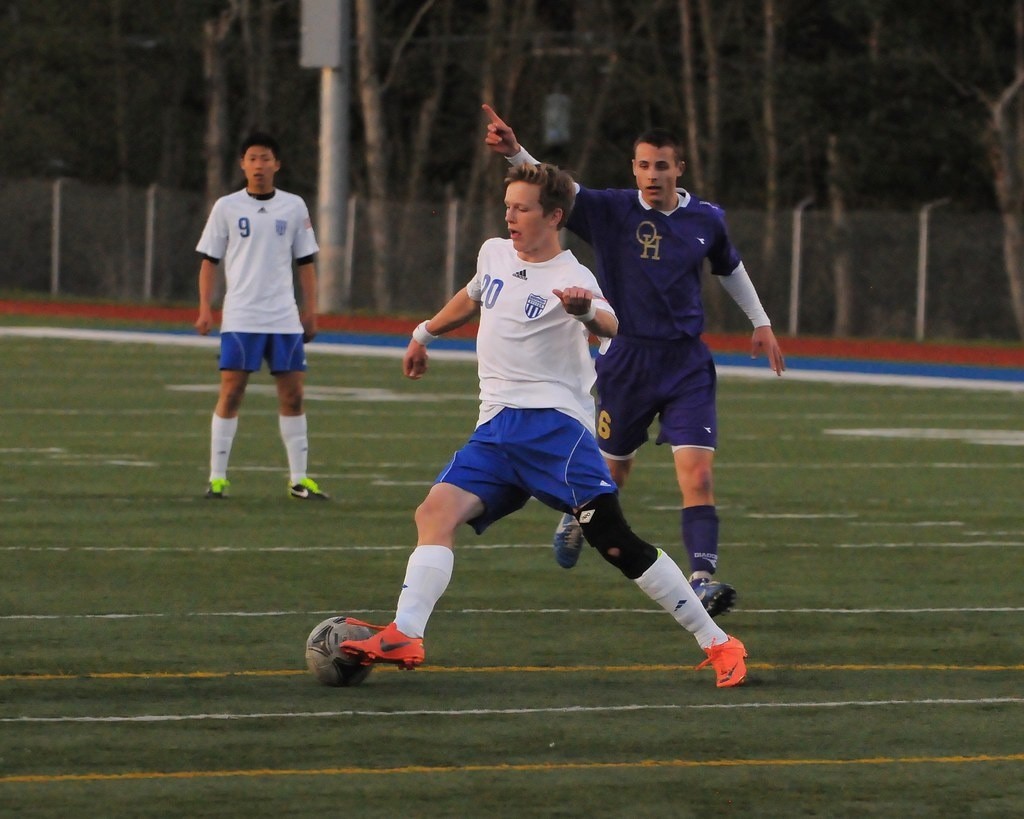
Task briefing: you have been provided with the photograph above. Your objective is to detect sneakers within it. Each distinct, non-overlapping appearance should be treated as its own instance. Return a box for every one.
[338,617,426,670]
[207,478,230,498]
[553,513,585,568]
[694,634,748,688]
[690,577,736,618]
[288,477,330,501]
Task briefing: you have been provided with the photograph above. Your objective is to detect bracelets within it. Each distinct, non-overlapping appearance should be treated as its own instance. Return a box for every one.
[571,304,596,323]
[412,320,438,345]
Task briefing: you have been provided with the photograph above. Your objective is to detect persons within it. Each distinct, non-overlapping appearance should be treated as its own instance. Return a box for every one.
[337,168,748,687]
[194,134,332,500]
[483,104,786,619]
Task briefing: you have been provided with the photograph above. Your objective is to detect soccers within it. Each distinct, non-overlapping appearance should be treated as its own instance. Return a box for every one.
[303,615,374,687]
